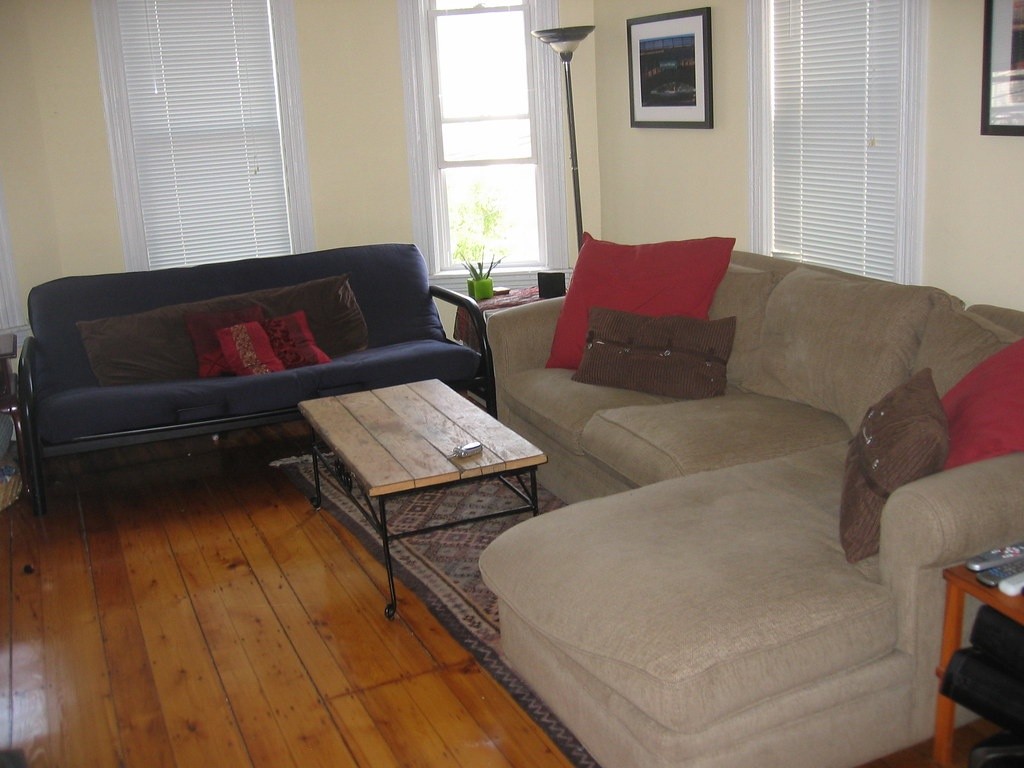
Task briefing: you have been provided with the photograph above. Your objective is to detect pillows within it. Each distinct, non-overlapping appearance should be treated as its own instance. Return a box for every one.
[935,336,1024,468]
[76,272,369,387]
[571,308,737,398]
[841,368,950,563]
[216,310,331,378]
[183,303,263,376]
[545,231,735,368]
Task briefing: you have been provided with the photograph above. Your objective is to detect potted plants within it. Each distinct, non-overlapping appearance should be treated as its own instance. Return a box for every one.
[458,247,506,301]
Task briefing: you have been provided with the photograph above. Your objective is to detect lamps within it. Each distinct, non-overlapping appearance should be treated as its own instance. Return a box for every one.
[532,26,596,251]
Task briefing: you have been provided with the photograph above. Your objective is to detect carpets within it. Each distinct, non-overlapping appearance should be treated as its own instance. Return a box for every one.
[274,452,599,768]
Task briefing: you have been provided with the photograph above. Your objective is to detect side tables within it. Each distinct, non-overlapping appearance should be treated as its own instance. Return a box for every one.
[931,564,1024,768]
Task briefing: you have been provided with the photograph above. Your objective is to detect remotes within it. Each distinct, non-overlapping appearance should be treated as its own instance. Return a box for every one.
[965,541,1024,597]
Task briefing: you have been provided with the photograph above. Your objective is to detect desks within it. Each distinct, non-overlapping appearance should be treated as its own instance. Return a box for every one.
[299,380,547,619]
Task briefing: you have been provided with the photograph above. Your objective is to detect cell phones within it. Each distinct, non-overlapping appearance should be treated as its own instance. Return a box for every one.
[446,442,482,459]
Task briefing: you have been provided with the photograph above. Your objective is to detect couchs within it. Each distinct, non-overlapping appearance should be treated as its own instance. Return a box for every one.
[476,248,1024,768]
[11,243,497,517]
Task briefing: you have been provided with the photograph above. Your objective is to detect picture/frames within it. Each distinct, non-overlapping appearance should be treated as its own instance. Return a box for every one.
[627,6,712,129]
[980,0,1024,138]
